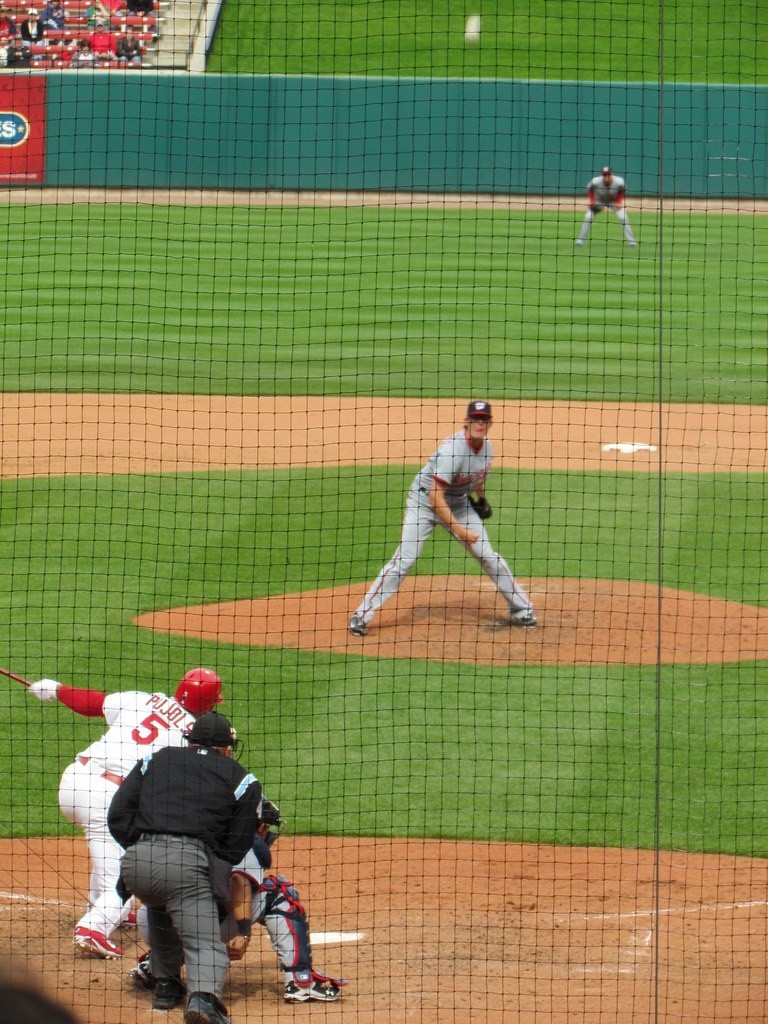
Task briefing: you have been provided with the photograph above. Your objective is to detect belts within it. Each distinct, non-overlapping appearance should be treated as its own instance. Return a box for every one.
[420,487,426,492]
[138,832,207,850]
[77,756,126,786]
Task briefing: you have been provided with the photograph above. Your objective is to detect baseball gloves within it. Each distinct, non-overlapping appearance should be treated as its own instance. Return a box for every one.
[468,495,492,519]
[590,205,601,212]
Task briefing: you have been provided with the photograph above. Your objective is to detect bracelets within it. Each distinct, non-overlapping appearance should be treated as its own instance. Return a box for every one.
[237,919,251,936]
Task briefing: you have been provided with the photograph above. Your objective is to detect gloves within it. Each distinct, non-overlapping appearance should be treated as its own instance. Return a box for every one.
[28,679,62,701]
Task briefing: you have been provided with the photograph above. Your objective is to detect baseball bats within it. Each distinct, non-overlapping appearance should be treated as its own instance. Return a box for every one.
[0,667,32,687]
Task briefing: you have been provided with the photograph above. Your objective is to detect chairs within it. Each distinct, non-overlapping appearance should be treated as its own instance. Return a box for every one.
[0,0,170,69]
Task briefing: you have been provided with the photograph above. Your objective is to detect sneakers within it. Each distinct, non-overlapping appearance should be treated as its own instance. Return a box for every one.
[284,979,340,1003]
[152,976,187,1008]
[117,913,138,930]
[184,994,233,1024]
[348,612,368,635]
[128,960,157,992]
[71,926,125,960]
[519,606,537,624]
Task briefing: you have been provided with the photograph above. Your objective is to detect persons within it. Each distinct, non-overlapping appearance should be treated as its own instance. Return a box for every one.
[0,7,16,47]
[577,166,636,246]
[8,37,31,68]
[70,39,99,68]
[88,22,117,59]
[86,0,110,31]
[107,712,262,1024]
[21,8,44,60]
[41,0,69,45]
[349,400,537,636]
[117,30,142,61]
[127,0,154,17]
[129,794,350,1004]
[102,0,123,16]
[27,668,224,960]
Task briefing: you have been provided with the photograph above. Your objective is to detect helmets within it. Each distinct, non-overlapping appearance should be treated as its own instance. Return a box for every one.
[260,794,281,824]
[175,668,224,713]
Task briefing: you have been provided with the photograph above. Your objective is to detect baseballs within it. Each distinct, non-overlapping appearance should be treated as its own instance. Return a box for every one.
[466,16,480,43]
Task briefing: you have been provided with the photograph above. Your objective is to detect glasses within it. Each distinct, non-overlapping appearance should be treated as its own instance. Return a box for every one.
[468,416,490,424]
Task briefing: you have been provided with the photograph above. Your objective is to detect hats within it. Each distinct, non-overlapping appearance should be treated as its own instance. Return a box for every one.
[603,167,611,175]
[96,21,104,27]
[467,401,493,417]
[14,34,22,39]
[28,8,38,15]
[187,711,233,748]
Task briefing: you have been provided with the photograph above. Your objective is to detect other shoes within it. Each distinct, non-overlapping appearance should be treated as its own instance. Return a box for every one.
[628,242,637,247]
[577,238,584,246]
